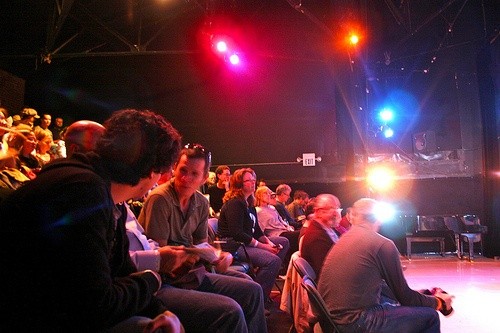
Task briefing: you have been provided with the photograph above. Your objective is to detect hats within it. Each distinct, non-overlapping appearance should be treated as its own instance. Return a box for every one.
[22,108,41,119]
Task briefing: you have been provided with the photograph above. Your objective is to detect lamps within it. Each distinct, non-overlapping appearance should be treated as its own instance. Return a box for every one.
[297,153,322,166]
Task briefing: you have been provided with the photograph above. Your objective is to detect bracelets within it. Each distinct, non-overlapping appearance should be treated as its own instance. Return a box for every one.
[254,240,258,247]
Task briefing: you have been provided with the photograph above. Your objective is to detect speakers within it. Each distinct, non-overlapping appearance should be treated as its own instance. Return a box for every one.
[412,130,436,151]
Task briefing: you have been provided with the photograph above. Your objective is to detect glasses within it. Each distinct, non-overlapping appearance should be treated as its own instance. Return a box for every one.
[184,144,210,164]
[243,179,256,183]
[319,208,343,212]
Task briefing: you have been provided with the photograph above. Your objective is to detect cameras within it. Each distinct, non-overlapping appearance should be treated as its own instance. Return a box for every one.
[439,291,453,316]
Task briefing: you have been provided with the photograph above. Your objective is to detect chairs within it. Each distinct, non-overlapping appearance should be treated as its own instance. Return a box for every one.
[288,254,339,333]
[206,216,282,304]
[399,213,488,264]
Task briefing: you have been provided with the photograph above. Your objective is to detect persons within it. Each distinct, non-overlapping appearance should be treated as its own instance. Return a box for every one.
[0,109,183,333]
[0,104,351,315]
[316,197,455,333]
[66,121,269,333]
[136,143,254,280]
